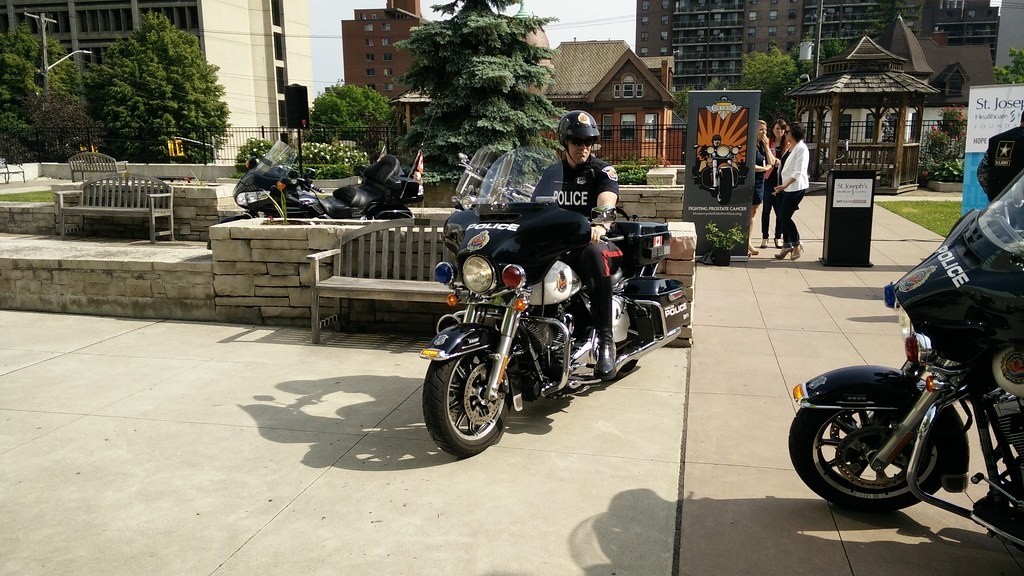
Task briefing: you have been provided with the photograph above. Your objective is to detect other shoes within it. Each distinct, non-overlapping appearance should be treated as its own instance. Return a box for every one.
[761,238,768,248]
[774,237,784,248]
[747,250,752,258]
[748,246,759,254]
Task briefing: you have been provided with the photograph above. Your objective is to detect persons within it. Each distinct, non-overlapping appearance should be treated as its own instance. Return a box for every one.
[748,120,775,256]
[760,120,790,249]
[772,123,809,260]
[976,111,1024,203]
[528,110,619,381]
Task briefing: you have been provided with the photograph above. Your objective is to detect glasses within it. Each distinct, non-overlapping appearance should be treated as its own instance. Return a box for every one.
[566,136,594,147]
[784,131,791,135]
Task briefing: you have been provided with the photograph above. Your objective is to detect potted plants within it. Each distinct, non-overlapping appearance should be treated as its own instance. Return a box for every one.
[705,219,749,267]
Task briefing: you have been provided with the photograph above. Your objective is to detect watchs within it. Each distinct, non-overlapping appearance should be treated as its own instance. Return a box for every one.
[596,223,610,232]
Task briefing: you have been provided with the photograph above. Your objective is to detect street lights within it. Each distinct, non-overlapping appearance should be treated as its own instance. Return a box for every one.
[43,50,92,103]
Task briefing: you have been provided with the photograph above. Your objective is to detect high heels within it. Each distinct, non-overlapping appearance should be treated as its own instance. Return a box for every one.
[791,245,804,260]
[775,247,793,260]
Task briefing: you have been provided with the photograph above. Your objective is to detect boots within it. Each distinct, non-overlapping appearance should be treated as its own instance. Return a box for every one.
[585,275,616,381]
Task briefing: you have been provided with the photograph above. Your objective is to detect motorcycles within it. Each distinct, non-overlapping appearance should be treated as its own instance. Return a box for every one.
[419,144,691,459]
[219,141,420,224]
[789,170,1024,550]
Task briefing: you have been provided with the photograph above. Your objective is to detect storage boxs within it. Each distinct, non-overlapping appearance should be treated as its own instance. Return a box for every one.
[398,176,419,197]
[607,221,672,268]
[624,276,692,337]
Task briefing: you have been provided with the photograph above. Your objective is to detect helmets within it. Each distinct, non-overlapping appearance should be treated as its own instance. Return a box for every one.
[557,110,600,144]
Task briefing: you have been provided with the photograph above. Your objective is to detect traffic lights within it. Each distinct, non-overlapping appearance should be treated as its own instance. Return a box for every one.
[176,140,184,156]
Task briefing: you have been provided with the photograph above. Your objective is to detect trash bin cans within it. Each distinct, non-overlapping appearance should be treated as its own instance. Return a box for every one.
[647,168,677,186]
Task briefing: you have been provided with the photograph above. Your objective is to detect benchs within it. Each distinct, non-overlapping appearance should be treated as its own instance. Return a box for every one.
[0,158,25,184]
[67,152,129,183]
[306,218,469,344]
[54,173,174,244]
